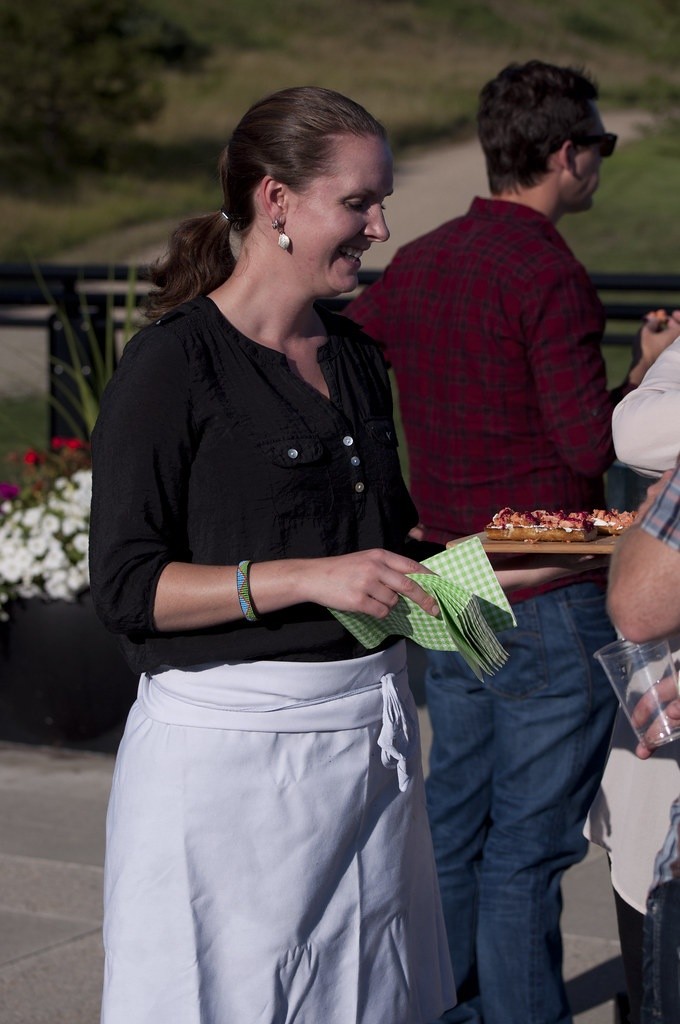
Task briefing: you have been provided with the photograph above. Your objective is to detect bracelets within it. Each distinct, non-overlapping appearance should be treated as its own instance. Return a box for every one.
[236,561,258,622]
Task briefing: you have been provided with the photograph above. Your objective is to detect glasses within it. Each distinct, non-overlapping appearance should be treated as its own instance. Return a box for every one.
[548,132,617,158]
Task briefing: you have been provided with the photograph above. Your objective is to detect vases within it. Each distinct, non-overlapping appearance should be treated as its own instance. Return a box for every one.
[0,589,141,741]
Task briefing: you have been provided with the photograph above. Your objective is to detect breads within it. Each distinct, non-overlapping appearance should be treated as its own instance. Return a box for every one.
[486,507,598,542]
[588,509,639,536]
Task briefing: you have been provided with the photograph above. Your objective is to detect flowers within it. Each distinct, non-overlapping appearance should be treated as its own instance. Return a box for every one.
[1,434,150,614]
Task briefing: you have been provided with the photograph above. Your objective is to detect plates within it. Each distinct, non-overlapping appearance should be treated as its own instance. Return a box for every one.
[446,530,621,552]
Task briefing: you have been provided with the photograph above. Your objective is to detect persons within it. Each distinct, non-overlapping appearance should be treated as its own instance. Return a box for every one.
[581,310,680,1024]
[337,59,680,1024]
[89,84,611,1024]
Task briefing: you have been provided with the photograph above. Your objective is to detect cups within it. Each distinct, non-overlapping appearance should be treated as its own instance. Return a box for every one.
[592,637,680,749]
